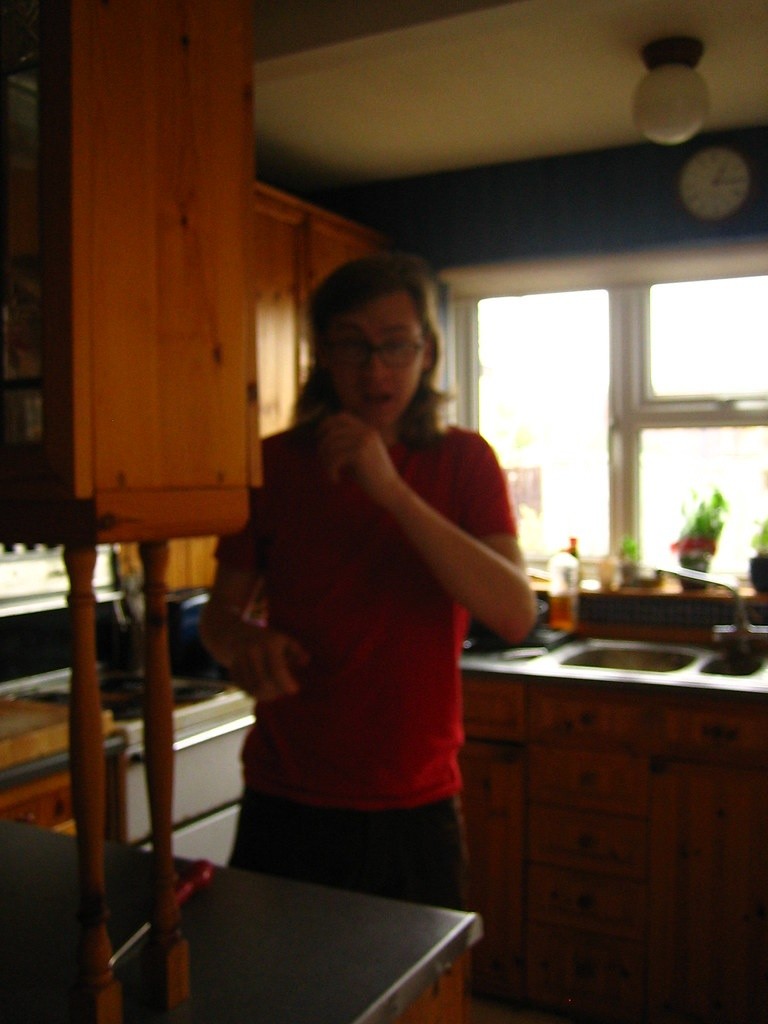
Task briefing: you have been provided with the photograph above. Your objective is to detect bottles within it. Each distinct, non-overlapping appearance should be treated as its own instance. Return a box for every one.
[549,546,577,632]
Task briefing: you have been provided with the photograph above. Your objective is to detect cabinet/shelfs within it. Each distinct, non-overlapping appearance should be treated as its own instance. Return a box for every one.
[461,674,768,1024]
[0,0,262,553]
[112,178,381,589]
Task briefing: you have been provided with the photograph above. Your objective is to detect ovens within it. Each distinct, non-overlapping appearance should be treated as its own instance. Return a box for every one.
[127,712,256,867]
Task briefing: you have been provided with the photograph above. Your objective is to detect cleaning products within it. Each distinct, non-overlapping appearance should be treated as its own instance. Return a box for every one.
[543,538,581,629]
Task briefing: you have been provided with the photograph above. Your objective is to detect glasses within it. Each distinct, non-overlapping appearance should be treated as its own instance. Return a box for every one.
[323,339,426,369]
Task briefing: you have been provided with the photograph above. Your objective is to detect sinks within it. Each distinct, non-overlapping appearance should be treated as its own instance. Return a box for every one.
[558,635,698,674]
[697,640,767,679]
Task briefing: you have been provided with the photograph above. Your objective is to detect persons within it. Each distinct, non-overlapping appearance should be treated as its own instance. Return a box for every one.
[195,249,540,910]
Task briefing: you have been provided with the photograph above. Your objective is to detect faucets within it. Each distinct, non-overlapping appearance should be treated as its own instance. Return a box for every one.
[652,562,768,644]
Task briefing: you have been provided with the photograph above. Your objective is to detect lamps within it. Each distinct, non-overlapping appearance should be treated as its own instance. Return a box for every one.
[632,37,709,146]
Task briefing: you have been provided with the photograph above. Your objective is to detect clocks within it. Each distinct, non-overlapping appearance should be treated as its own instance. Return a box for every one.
[678,150,751,226]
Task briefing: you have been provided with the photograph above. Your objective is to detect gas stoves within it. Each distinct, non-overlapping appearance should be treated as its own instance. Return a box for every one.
[0,590,253,745]
